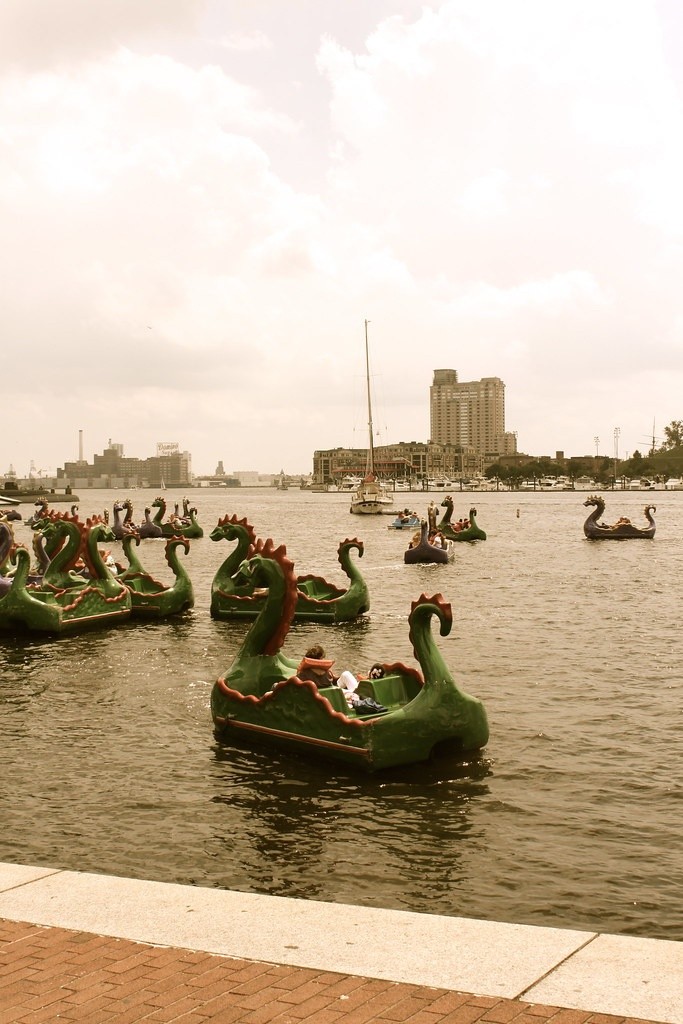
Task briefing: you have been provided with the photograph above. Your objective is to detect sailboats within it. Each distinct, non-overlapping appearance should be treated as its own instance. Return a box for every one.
[349,318,395,515]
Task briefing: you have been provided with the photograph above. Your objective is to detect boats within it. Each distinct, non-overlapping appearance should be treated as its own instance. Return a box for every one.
[212,513,371,624]
[404,500,455,565]
[583,495,658,540]
[435,495,487,541]
[387,513,424,530]
[0,495,204,635]
[209,537,490,773]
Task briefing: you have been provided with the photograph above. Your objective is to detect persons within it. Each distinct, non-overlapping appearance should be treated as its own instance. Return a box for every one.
[122,517,140,529]
[168,513,184,525]
[398,511,404,520]
[86,514,103,527]
[453,518,470,529]
[77,550,118,574]
[414,529,445,547]
[413,512,418,518]
[301,646,384,698]
[40,509,69,520]
